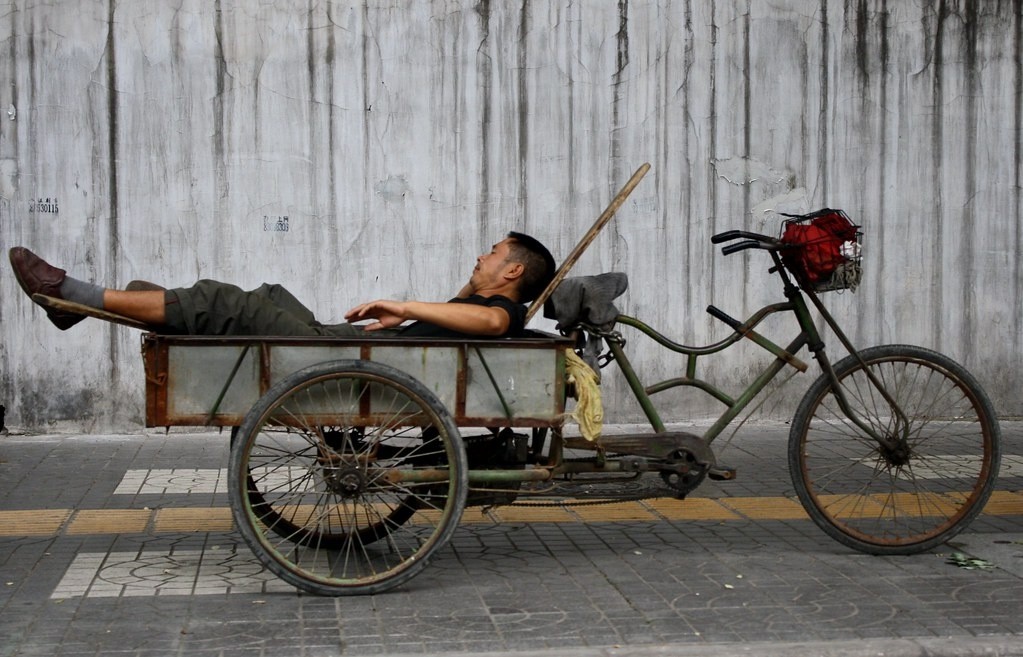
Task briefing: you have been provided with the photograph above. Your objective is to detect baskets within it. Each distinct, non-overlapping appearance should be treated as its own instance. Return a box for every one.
[779,208,864,293]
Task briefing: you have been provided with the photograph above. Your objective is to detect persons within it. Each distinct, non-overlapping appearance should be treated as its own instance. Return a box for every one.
[9,231,556,336]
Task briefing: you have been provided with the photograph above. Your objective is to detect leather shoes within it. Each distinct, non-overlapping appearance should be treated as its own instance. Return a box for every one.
[125,280,167,291]
[9,246,88,331]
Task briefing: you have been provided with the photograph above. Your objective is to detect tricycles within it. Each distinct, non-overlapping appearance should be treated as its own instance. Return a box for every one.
[141,208,1002,597]
